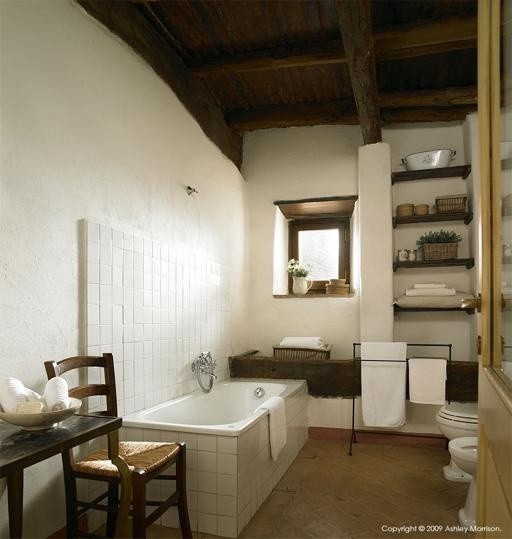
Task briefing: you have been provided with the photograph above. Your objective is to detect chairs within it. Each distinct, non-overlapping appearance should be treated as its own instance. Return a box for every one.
[43,352,193,539]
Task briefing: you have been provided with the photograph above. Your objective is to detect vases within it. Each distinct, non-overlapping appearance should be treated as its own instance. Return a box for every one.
[293,277,313,294]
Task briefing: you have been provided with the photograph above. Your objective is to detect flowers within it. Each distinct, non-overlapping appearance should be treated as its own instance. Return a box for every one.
[286,258,316,276]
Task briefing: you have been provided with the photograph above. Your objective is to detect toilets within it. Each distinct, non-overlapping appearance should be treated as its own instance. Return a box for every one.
[445,435,480,533]
[433,399,479,484]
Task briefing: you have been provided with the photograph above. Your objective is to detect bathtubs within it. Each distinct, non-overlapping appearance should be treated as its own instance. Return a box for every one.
[120,377,308,539]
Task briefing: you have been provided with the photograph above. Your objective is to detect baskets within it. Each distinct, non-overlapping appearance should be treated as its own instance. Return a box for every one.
[273,347,330,359]
[435,194,467,215]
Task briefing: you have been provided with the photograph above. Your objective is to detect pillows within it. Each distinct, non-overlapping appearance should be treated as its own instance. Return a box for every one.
[392,291,477,308]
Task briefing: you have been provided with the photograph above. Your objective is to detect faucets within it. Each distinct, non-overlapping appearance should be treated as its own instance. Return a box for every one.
[192,353,218,380]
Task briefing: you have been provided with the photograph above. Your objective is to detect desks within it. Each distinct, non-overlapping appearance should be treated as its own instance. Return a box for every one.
[0,410,122,539]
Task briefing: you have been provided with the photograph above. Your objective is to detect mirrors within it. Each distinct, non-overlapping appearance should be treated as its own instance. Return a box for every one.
[478,1,512,388]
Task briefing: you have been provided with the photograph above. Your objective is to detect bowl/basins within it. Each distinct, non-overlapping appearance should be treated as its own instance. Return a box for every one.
[0,398,82,432]
[400,149,457,172]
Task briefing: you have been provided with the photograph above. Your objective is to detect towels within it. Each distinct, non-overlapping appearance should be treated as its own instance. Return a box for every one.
[409,357,448,404]
[255,395,286,463]
[406,288,456,297]
[45,376,71,412]
[1,378,41,415]
[280,337,326,353]
[414,283,448,288]
[361,344,407,430]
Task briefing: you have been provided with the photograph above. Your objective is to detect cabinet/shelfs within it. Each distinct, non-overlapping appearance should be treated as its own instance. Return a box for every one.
[391,162,478,315]
[501,157,511,317]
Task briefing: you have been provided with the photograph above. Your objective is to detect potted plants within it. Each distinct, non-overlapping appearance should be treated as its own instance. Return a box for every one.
[417,231,462,260]
